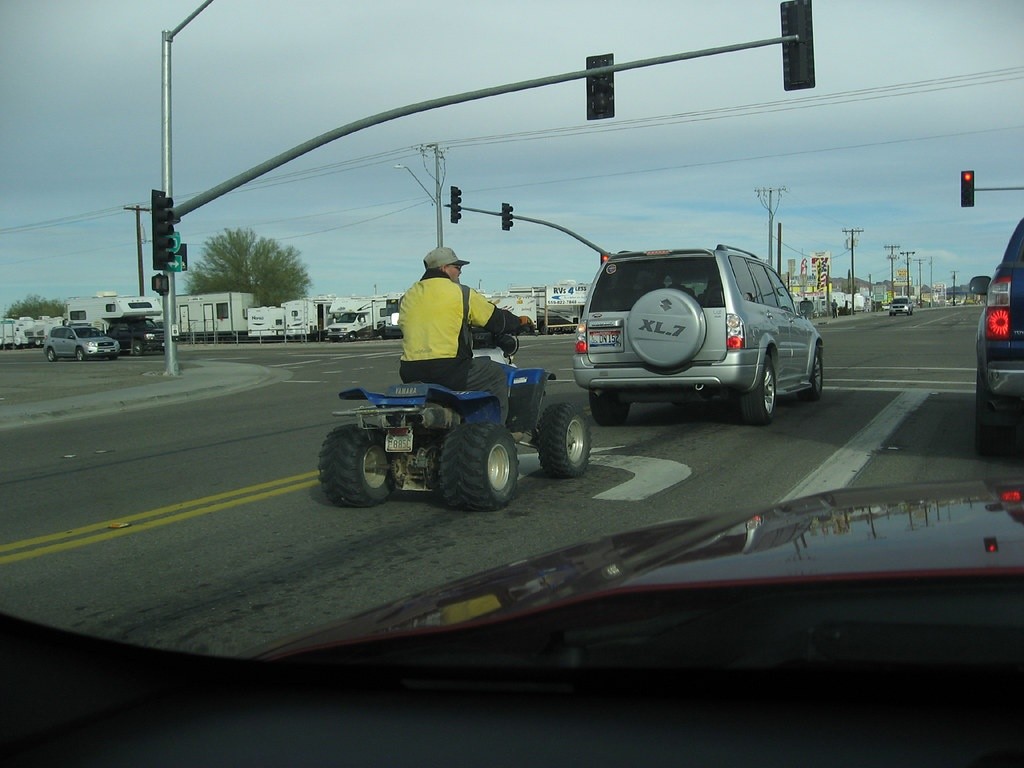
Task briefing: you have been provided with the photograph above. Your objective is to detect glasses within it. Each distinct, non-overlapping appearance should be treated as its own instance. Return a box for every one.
[449,264,462,270]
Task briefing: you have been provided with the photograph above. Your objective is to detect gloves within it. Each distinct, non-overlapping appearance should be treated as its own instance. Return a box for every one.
[519,315,534,331]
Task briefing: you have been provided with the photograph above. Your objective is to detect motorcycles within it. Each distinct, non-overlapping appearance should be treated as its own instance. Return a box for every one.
[317,324,593,513]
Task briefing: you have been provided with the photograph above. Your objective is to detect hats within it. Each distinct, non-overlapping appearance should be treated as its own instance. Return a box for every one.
[423,247,470,270]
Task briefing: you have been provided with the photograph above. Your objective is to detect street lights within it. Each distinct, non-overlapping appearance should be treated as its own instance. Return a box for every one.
[392,163,443,249]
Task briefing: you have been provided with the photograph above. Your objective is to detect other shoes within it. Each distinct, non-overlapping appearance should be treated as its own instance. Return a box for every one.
[510,431,523,441]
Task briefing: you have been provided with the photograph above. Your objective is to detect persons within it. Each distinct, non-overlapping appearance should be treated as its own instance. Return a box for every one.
[397,248,534,429]
[831,299,838,318]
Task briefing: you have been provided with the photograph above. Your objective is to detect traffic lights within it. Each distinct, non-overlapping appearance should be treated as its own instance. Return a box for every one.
[152,189,175,271]
[960,170,974,208]
[152,275,168,291]
[450,185,463,223]
[502,203,514,232]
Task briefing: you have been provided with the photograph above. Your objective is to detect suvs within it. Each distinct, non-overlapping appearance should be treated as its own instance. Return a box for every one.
[968,217,1024,455]
[44,324,120,363]
[888,297,913,316]
[574,243,824,427]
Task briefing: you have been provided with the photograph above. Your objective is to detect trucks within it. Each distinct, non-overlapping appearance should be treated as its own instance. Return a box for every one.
[247,286,584,344]
[65,291,166,357]
[0,313,68,350]
